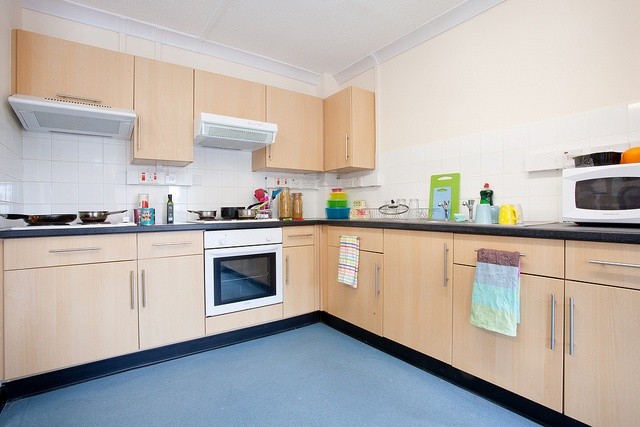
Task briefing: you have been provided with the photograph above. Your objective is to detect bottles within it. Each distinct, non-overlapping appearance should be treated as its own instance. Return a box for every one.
[167,194,174,223]
[289,192,303,219]
[279,186,291,219]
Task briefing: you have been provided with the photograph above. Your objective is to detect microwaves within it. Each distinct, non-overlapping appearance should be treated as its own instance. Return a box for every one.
[560,161,640,226]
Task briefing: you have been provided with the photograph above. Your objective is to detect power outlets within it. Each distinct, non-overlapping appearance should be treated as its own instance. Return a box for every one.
[275,177,291,187]
[138,172,159,184]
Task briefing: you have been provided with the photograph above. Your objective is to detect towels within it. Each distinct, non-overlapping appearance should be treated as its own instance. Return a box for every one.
[469,248,522,336]
[336,235,361,289]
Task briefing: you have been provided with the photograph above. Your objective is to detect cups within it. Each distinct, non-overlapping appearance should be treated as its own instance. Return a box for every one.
[490,205,499,224]
[474,203,492,225]
[396,198,407,218]
[408,198,420,221]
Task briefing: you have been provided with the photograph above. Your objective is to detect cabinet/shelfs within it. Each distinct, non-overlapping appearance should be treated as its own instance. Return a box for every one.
[452,232,640,427]
[10,28,135,113]
[282,223,323,318]
[130,54,194,168]
[133,228,205,352]
[384,228,453,365]
[205,301,283,337]
[323,222,384,339]
[252,81,324,174]
[1,231,138,384]
[321,86,376,174]
[194,67,266,123]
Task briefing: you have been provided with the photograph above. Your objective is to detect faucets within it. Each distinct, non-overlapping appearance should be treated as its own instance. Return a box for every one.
[438,199,451,219]
[462,198,475,220]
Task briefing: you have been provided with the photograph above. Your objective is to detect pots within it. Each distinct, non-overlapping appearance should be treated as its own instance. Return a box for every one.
[220,201,263,218]
[0,211,77,226]
[187,207,217,219]
[77,209,128,223]
[237,200,269,218]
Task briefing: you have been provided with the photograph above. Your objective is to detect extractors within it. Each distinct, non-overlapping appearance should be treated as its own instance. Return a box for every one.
[8,93,136,140]
[194,112,279,152]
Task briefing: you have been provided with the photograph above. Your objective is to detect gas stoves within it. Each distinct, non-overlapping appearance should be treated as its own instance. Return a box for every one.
[10,222,137,231]
[188,217,281,225]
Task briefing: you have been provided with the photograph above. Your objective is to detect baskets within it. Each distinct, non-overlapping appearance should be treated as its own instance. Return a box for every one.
[361,208,430,220]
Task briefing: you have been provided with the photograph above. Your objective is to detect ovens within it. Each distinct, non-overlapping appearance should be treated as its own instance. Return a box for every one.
[204,227,284,318]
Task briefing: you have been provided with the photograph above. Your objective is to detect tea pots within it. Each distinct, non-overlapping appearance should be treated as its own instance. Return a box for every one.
[498,203,520,225]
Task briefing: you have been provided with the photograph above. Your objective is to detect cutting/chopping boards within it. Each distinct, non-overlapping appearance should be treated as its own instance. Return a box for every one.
[427,173,460,221]
[432,186,451,220]
